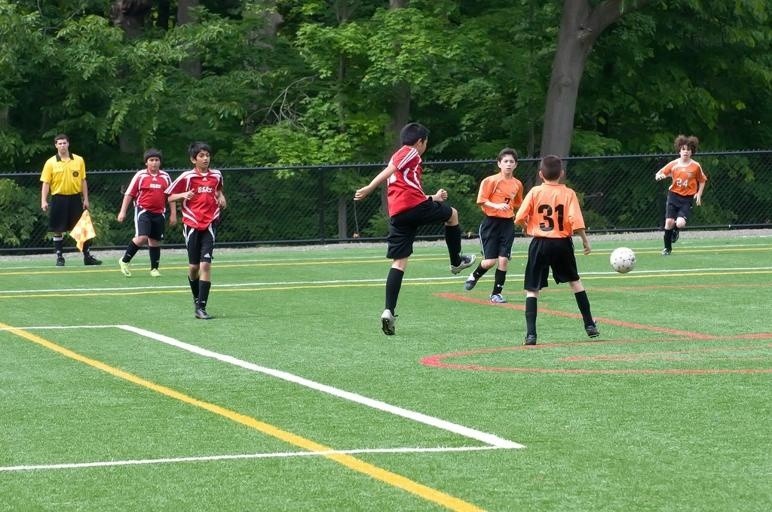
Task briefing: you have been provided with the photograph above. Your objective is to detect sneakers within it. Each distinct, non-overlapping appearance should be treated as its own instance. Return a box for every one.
[84,256,102,265]
[119,257,131,278]
[465,269,479,290]
[661,247,672,256]
[586,320,600,338]
[195,307,211,318]
[380,309,398,335]
[489,293,506,303]
[450,252,477,275]
[671,221,679,243]
[56,258,65,266]
[150,268,161,277]
[522,334,537,345]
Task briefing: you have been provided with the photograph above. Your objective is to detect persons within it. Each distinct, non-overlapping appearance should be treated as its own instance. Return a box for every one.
[164,142,227,320]
[514,155,600,345]
[39,135,103,266]
[354,123,476,335]
[655,135,707,255]
[465,148,523,304]
[117,150,177,277]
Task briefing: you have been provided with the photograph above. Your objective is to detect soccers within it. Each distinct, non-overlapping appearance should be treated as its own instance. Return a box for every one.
[610,247,636,273]
